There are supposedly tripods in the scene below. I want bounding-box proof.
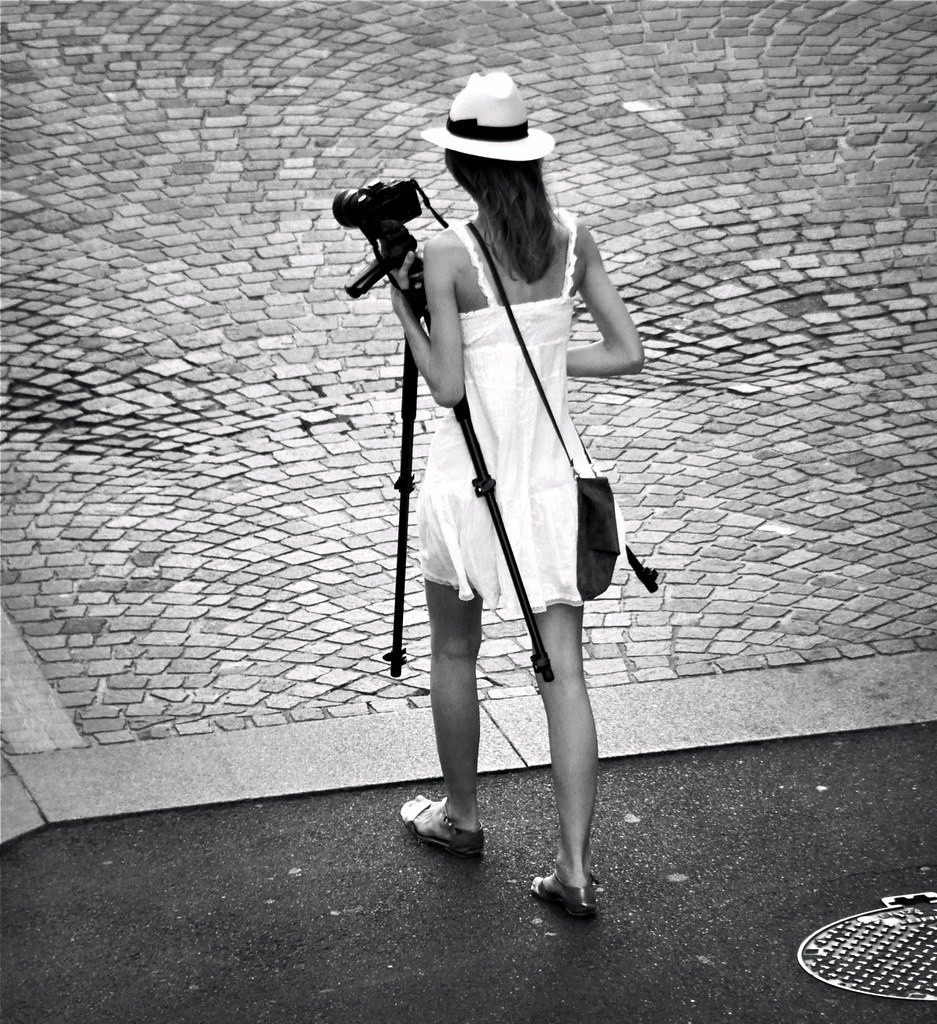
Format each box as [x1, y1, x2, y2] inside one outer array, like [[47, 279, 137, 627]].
[[345, 219, 658, 684]]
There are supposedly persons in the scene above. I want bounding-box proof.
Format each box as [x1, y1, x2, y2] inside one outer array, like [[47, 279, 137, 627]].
[[391, 71, 642, 919]]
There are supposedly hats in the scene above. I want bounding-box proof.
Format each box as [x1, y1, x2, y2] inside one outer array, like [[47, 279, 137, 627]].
[[420, 71, 555, 162]]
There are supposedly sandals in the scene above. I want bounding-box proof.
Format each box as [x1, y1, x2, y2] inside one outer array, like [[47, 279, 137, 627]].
[[401, 796, 483, 857], [532, 869, 601, 913]]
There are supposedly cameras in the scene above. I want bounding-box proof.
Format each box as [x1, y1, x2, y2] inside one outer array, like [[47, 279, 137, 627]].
[[331, 178, 423, 240]]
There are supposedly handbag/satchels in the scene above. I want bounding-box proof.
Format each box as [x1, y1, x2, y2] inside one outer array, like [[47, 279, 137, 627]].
[[577, 477, 621, 601]]
